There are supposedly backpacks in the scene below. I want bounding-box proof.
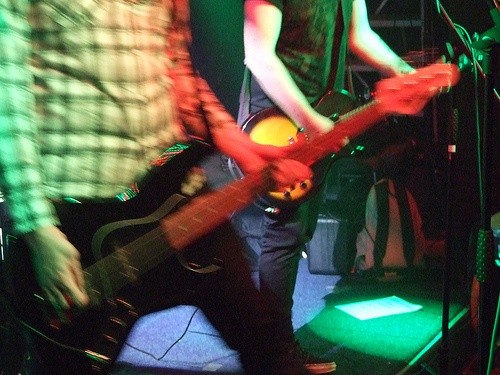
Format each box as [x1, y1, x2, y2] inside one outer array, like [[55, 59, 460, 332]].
[[353, 175, 427, 276]]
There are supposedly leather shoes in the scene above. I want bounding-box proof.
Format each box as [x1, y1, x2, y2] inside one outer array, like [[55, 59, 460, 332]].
[[287, 343, 337, 373]]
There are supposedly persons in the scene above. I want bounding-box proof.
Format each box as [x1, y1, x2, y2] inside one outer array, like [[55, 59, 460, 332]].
[[355, 173, 447, 285], [236, 0, 417, 375], [0, 0, 313, 375], [470, 212, 500, 346]]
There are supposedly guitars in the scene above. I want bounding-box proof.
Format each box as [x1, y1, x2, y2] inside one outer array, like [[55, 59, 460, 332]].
[[0, 64, 460, 375], [228, 56, 452, 214]]
